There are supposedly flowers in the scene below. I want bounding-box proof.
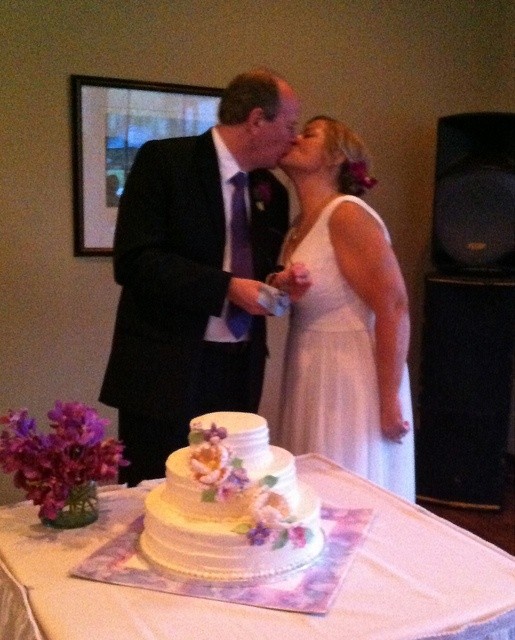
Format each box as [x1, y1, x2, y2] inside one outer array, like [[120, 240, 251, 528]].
[[0, 399, 131, 521], [187, 422, 314, 552]]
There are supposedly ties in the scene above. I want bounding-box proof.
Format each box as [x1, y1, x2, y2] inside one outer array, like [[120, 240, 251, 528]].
[[225, 171, 256, 340]]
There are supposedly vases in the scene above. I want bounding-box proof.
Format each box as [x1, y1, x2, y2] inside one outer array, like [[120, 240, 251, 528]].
[[45, 480, 99, 528]]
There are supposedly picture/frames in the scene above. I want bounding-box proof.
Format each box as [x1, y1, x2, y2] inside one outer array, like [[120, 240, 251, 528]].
[[69, 73, 226, 255]]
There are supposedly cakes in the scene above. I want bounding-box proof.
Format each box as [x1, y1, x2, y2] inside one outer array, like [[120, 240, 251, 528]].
[[137, 411, 328, 583]]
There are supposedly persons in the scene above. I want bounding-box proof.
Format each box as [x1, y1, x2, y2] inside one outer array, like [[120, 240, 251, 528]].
[[277, 115, 416, 506], [98, 69, 303, 487]]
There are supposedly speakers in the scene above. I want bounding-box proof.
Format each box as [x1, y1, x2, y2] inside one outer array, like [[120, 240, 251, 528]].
[[428, 110, 514, 276], [414, 272, 515, 514]]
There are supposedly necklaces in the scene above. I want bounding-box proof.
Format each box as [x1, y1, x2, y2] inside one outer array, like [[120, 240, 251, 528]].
[[292, 193, 337, 241]]
